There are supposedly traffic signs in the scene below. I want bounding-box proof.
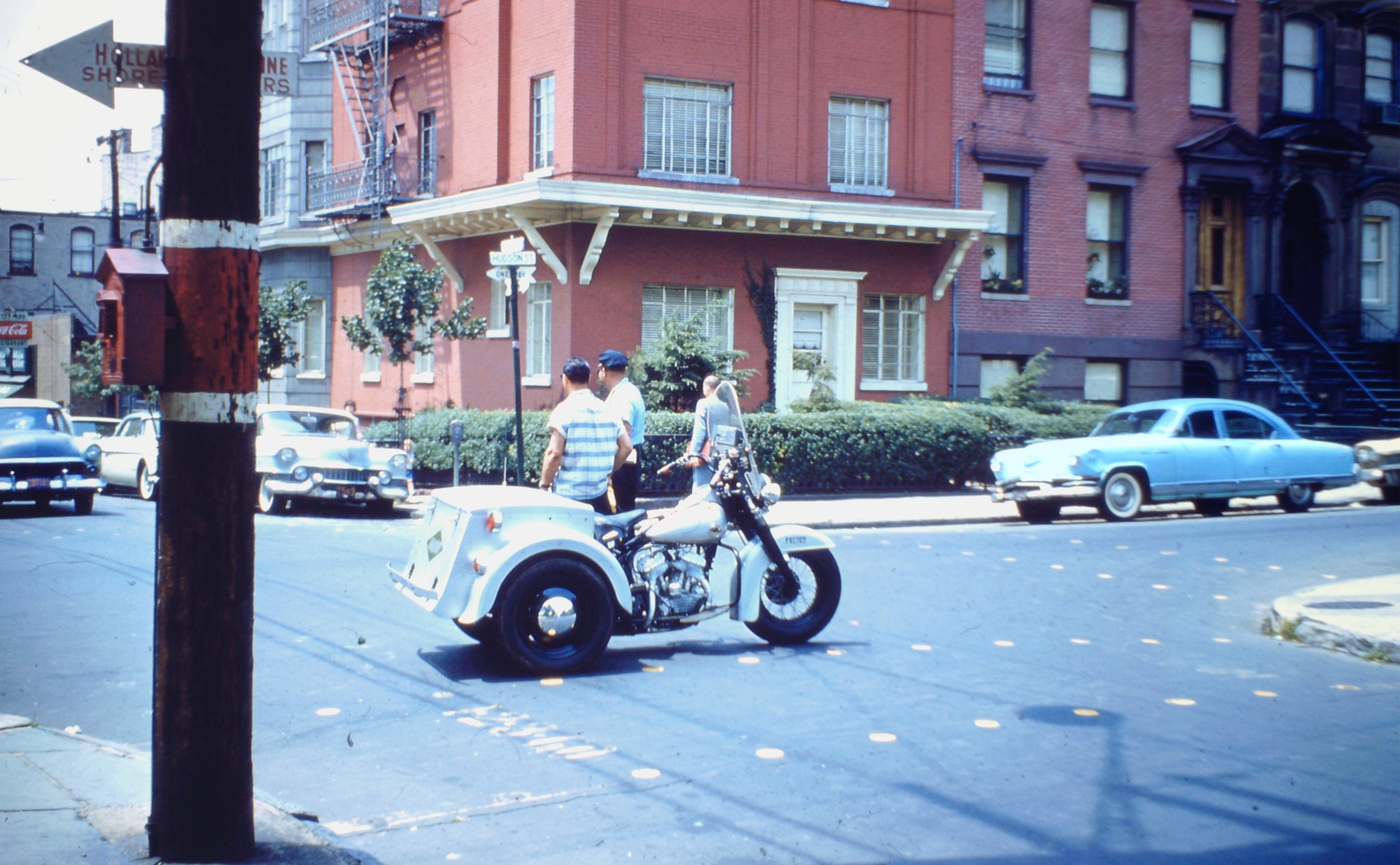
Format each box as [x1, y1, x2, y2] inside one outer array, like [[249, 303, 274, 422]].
[[486, 265, 537, 285], [19, 19, 302, 109]]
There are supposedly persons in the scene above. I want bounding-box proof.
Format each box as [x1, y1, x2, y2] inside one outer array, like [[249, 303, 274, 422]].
[[330, 399, 363, 439], [540, 356, 633, 540], [689, 375, 730, 493], [400, 438, 416, 501], [598, 349, 645, 547]]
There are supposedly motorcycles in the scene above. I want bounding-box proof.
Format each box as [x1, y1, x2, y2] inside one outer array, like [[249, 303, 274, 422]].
[[387, 378, 842, 679]]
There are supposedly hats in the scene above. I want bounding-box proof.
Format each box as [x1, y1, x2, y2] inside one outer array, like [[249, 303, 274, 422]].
[[598, 349, 628, 369]]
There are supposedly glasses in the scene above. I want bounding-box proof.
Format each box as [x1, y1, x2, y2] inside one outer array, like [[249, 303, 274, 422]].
[[560, 372, 565, 380]]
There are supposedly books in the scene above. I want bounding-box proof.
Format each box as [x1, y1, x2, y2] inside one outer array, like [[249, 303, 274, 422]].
[[683, 439, 711, 467]]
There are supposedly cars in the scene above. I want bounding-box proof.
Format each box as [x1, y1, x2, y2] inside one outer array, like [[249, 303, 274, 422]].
[[250, 404, 412, 513], [0, 397, 162, 516], [988, 398, 1399, 523]]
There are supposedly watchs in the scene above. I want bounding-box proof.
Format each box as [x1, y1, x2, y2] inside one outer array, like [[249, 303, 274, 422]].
[[539, 481, 550, 488]]
